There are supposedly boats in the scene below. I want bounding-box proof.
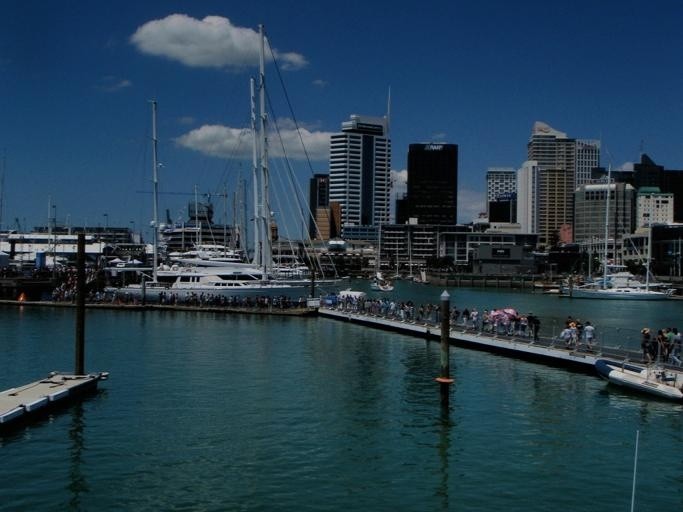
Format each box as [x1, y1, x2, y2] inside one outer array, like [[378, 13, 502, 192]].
[[594, 357, 683, 401], [367, 276, 396, 293]]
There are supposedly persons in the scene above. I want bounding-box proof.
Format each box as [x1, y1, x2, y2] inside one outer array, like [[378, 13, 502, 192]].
[[52, 266, 134, 306], [341, 295, 539, 341], [559, 317, 594, 352], [642, 327, 682, 368], [159, 291, 312, 310]]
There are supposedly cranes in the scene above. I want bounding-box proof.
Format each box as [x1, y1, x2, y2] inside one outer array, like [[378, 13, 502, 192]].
[[129, 180, 229, 203]]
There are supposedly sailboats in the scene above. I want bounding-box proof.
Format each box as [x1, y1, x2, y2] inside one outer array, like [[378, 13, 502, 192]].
[[103, 20, 350, 302], [567, 161, 671, 303], [0, 194, 112, 268]]
